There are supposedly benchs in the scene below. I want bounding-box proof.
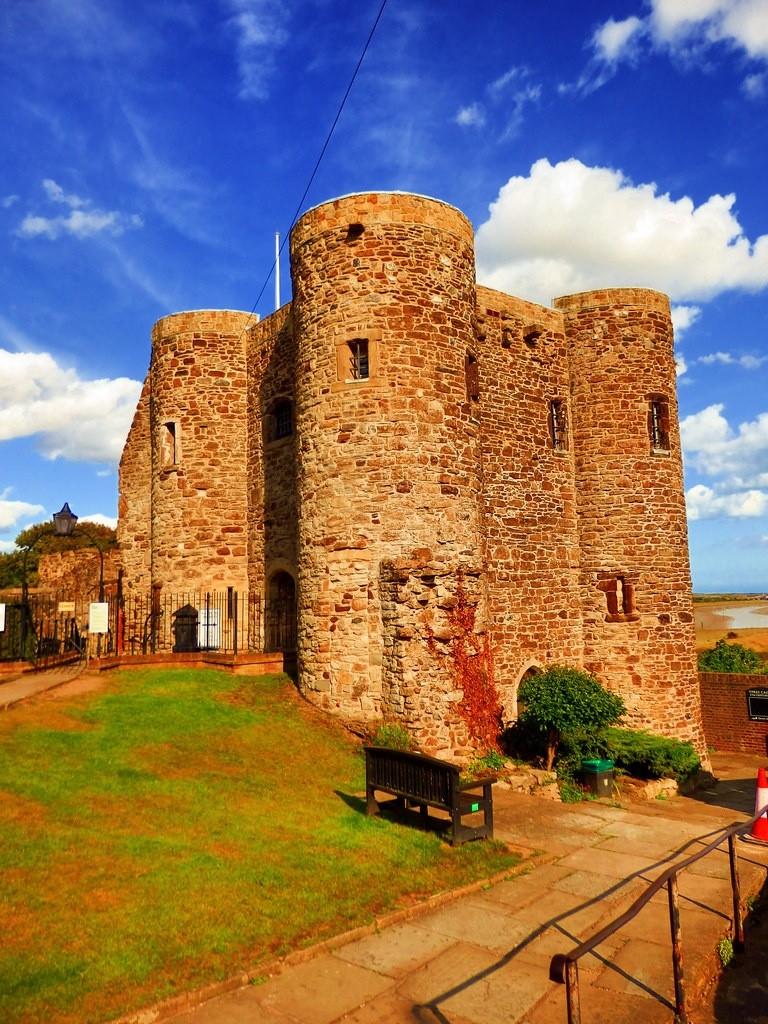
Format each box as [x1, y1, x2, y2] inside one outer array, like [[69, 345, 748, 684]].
[[364, 746, 499, 848]]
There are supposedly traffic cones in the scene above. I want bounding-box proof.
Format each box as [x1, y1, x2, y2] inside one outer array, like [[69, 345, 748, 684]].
[[738, 767, 768, 846]]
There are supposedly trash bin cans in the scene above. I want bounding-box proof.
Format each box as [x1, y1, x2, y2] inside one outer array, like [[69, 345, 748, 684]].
[[86, 624, 110, 655], [171, 604, 201, 652], [583, 759, 616, 799]]
